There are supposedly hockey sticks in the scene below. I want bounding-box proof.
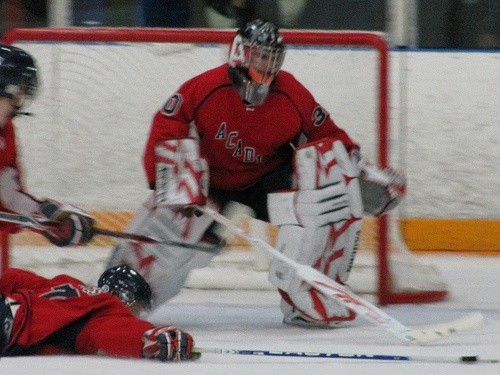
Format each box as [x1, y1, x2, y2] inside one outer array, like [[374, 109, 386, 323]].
[[192, 348, 500, 363], [0, 209, 225, 257], [192, 205, 483, 346]]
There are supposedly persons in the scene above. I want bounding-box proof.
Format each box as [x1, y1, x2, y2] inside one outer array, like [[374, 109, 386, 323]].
[[105, 18, 406, 329], [0, 44, 199, 361]]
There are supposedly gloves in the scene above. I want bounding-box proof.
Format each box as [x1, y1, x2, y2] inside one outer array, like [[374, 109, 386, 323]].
[[139, 323, 192, 361], [32, 198, 95, 245]]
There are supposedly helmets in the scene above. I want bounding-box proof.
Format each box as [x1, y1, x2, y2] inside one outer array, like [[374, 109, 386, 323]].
[[227, 19, 284, 105], [0, 43, 37, 87], [97, 264, 151, 306]]
